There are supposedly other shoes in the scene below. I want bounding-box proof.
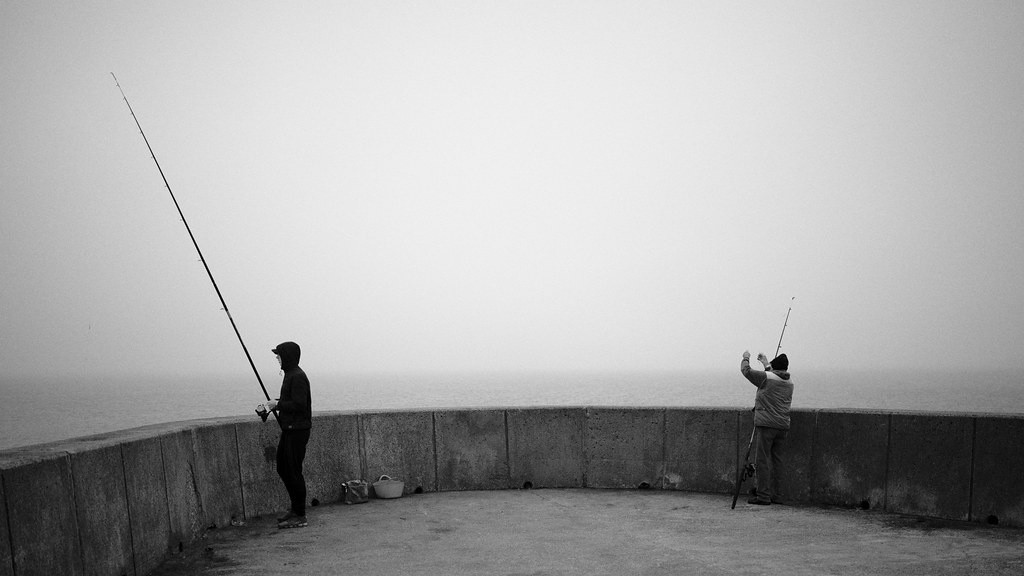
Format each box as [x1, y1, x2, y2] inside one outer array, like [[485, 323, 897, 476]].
[[747, 497, 770, 505]]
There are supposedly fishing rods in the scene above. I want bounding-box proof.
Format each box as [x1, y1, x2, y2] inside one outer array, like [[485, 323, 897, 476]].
[[731, 295, 795, 509], [110, 71, 283, 431]]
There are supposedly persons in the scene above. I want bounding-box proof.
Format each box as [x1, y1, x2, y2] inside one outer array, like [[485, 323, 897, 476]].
[[267, 341, 312, 526], [742, 349, 794, 505]]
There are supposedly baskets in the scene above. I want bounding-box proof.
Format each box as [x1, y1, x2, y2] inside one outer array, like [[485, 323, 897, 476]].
[[373, 474, 404, 498]]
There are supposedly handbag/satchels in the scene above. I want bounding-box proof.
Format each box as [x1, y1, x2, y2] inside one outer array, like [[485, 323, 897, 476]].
[[343, 480, 369, 504]]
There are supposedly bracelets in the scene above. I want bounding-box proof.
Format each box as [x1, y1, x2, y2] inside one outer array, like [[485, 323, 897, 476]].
[[276, 401, 279, 410]]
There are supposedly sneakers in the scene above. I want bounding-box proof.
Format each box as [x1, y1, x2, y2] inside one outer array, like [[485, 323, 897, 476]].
[[278, 515, 308, 528], [277, 511, 293, 521]]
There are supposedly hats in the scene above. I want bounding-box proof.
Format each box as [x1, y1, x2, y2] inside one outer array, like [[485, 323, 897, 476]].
[[770, 354, 788, 370], [272, 348, 279, 355]]
